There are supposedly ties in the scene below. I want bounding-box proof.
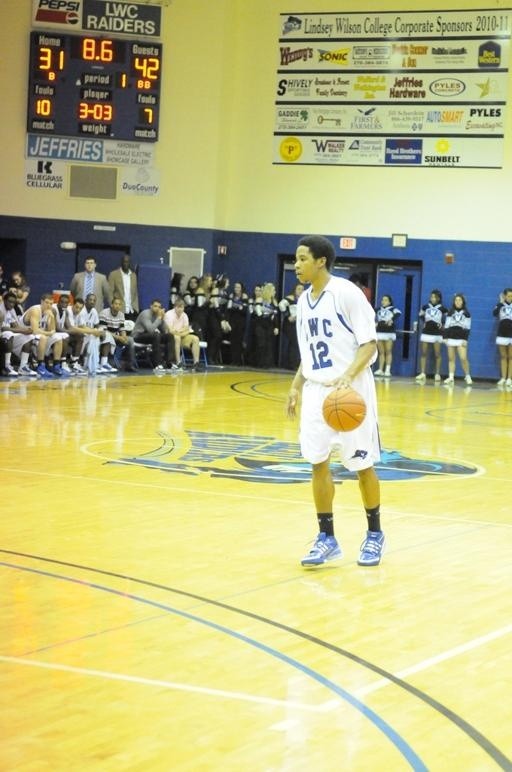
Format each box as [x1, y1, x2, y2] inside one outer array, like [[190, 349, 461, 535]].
[[85, 276, 92, 296]]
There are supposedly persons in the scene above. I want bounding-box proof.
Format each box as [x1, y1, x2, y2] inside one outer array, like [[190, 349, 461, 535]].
[[415, 290, 450, 381], [287, 236, 385, 567], [2, 255, 305, 376], [374, 294, 402, 377], [492, 288, 512, 386], [349, 273, 372, 304], [442, 294, 473, 385]]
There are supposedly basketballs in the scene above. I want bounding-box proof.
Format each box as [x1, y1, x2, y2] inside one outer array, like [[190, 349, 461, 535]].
[[324, 388, 364, 431]]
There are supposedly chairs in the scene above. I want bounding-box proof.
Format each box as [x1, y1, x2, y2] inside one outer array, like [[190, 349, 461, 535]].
[[111, 320, 210, 376]]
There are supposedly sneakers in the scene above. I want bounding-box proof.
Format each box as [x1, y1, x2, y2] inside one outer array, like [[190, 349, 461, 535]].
[[374, 367, 511, 386], [3, 361, 205, 376], [358, 531, 384, 566], [301, 531, 342, 568]]
